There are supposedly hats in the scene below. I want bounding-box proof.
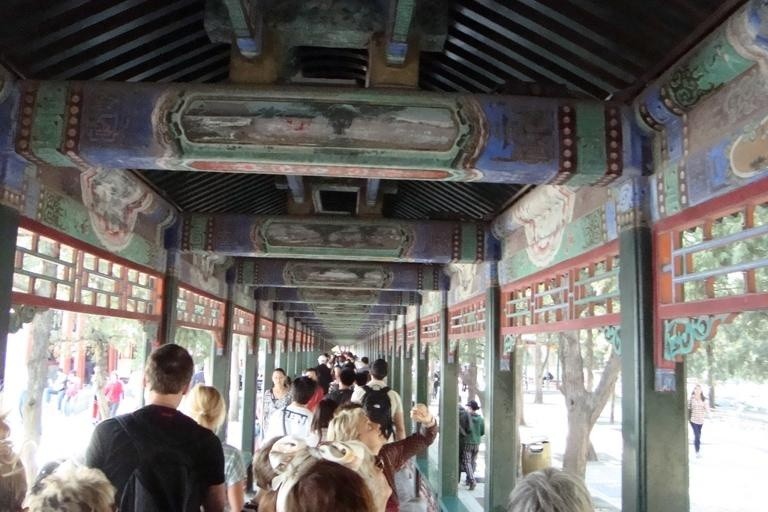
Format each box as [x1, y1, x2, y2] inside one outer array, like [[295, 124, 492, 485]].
[[466, 400, 478, 410]]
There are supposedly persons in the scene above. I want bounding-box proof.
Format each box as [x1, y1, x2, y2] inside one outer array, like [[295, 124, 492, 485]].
[[503, 467, 595, 512], [688, 385, 711, 458]]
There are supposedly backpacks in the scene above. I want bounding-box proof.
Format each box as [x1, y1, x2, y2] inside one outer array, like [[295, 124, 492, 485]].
[[116, 414, 203, 512], [361, 386, 391, 440]]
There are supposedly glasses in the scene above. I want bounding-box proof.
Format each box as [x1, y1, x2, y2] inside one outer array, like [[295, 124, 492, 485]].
[[371, 456, 384, 471]]
